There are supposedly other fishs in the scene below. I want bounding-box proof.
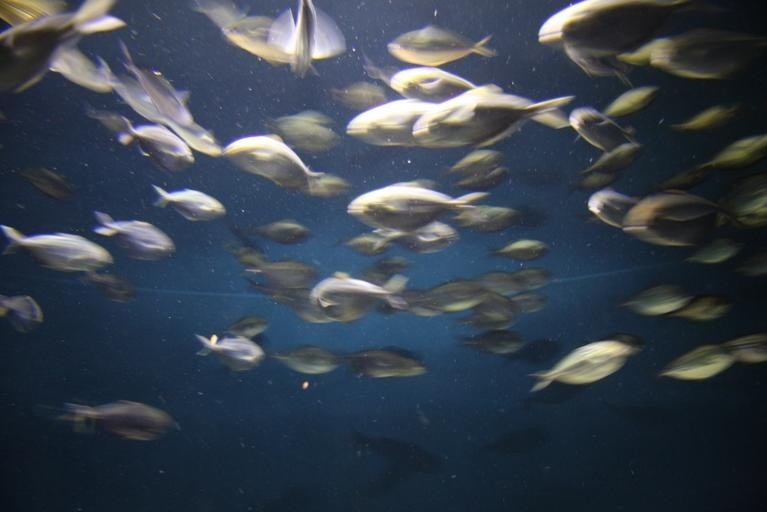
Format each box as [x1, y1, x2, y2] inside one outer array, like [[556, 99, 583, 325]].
[[55, 400, 183, 443], [0, 0, 767, 393]]
